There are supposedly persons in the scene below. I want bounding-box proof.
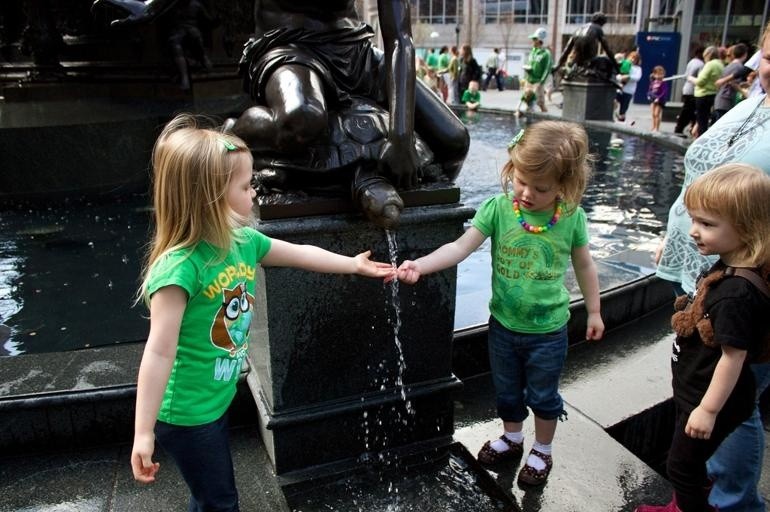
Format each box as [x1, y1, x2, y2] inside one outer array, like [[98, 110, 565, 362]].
[[629, 25, 770, 512], [128, 112, 397, 512], [219, 1, 469, 191], [383, 119, 606, 489], [371, 41, 385, 63], [669, 162, 770, 510], [552, 10, 621, 73]]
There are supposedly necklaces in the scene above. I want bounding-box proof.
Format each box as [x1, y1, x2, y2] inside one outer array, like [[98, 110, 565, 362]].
[[511, 194, 565, 234]]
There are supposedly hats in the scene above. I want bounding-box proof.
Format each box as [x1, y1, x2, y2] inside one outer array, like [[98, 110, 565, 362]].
[[614, 53, 625, 64], [528, 29, 546, 43]]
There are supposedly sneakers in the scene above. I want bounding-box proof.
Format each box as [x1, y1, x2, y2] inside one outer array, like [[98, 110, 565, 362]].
[[479, 436, 524, 463], [520, 448, 553, 485], [634, 491, 680, 512]]
